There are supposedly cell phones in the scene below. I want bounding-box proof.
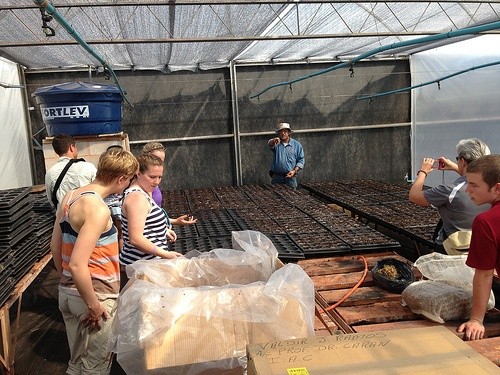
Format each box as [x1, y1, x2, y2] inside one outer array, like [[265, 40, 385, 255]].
[[431, 159, 443, 168]]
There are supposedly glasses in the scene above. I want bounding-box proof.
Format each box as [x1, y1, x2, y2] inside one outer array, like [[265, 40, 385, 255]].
[[456, 156, 463, 161]]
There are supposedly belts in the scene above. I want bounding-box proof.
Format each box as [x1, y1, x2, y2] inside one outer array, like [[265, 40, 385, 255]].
[[276, 172, 297, 176]]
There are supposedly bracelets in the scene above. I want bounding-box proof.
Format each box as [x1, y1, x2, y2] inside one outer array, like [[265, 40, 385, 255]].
[[416, 170, 428, 177]]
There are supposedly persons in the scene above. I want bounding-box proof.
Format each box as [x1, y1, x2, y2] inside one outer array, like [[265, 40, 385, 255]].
[[268, 122, 305, 190], [100, 144, 131, 253], [50, 145, 141, 375], [45, 134, 99, 214], [407, 137, 491, 264], [456, 154, 500, 341], [119, 151, 185, 288], [143, 141, 197, 226]]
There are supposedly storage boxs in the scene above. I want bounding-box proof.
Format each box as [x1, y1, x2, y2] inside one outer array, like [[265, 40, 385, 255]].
[[246, 326, 500, 375], [140, 257, 306, 375]]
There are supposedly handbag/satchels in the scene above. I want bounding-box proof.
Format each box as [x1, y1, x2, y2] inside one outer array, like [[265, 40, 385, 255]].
[[443, 230, 472, 255]]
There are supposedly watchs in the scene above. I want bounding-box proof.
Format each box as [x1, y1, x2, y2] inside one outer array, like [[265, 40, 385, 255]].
[[293, 169, 298, 173]]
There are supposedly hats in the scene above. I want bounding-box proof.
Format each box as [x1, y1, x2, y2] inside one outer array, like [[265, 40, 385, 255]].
[[275, 123, 292, 135]]
